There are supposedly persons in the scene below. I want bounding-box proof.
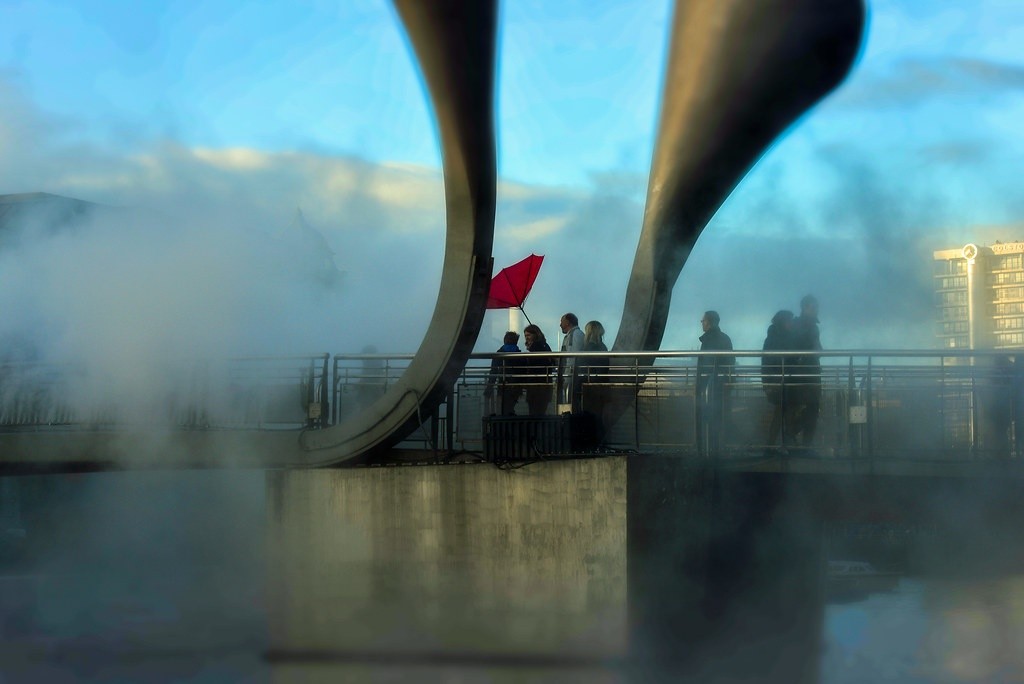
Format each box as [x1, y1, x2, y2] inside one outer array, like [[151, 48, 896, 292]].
[[761, 310, 806, 460], [522, 324, 556, 418], [353, 344, 386, 417], [482, 331, 526, 415], [695, 310, 736, 457], [581, 320, 611, 453], [558, 313, 584, 412], [793, 294, 824, 460]]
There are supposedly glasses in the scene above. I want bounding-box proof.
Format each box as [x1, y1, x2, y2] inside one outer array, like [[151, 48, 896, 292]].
[[701, 320, 705, 323]]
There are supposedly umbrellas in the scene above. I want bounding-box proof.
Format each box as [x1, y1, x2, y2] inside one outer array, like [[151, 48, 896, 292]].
[[486, 253, 546, 325]]
